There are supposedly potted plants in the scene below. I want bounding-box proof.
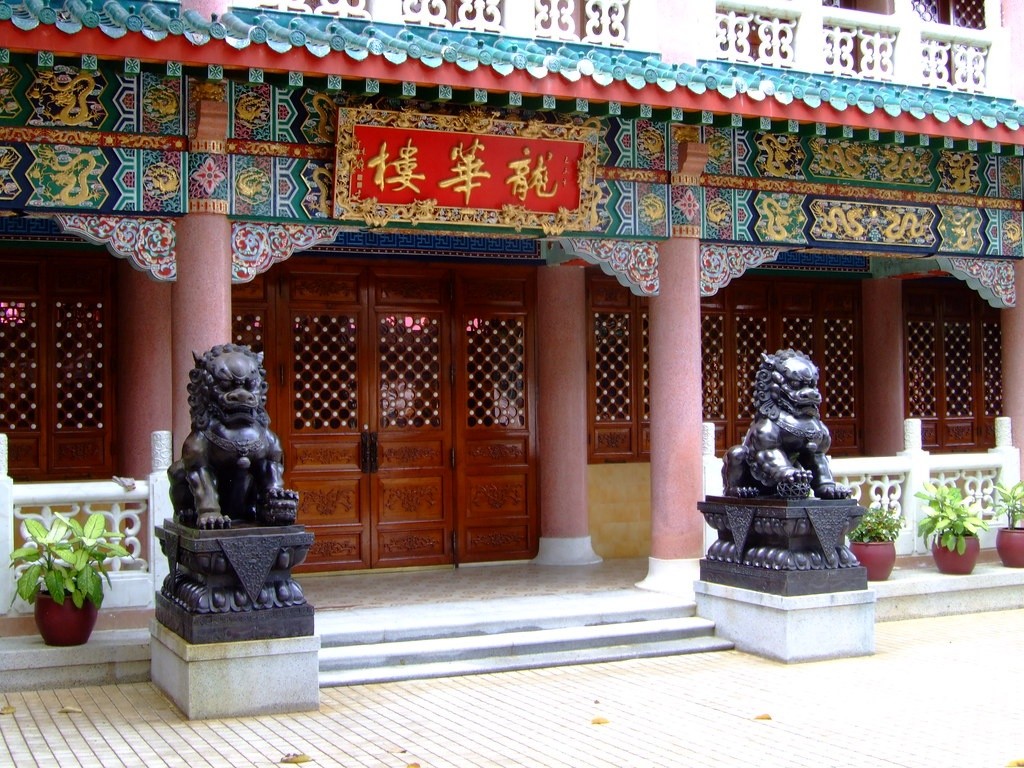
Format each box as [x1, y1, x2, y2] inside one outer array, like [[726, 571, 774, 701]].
[[985, 480, 1024, 568], [847, 506, 908, 580], [8, 511, 135, 648], [914, 480, 990, 575]]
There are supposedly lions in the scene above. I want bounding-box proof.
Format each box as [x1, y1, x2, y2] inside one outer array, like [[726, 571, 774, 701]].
[[721, 349, 853, 499], [168, 344, 299, 530]]
